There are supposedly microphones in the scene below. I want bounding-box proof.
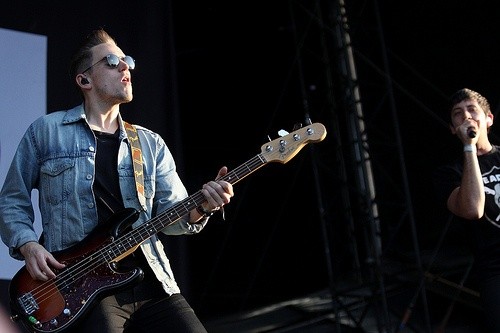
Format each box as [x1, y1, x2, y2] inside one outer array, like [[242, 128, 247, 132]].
[[467, 126, 476, 139]]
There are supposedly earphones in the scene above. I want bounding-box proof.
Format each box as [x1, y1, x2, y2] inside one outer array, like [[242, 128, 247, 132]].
[[81, 77, 89, 85]]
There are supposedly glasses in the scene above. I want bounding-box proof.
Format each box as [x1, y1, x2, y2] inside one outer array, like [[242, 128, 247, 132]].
[[81, 54, 135, 73]]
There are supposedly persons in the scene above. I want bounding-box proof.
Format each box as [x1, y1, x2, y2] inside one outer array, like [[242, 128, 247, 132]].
[[0, 31, 234, 333], [434, 88, 500, 333]]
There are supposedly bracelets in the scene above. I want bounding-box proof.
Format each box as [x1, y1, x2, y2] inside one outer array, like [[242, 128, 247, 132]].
[[196, 205, 212, 217], [463, 145, 478, 152]]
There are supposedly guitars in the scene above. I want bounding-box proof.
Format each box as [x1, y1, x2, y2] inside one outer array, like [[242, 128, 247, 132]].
[[7, 113, 326, 333]]
